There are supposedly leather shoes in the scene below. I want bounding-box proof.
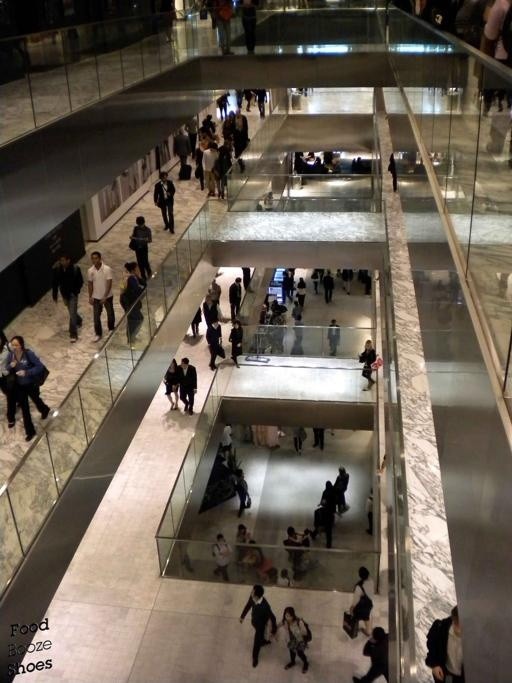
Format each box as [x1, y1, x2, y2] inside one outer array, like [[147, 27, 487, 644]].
[[9, 422, 15, 427], [42, 408, 50, 419], [26, 432, 35, 441], [185, 403, 193, 415]]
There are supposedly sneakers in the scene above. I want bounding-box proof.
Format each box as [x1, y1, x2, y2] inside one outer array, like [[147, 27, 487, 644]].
[[285, 662, 308, 673], [70, 337, 76, 342], [93, 335, 100, 342]]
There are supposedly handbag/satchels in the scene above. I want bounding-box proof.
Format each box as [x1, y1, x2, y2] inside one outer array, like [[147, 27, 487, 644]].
[[343, 595, 372, 639], [33, 365, 49, 386], [302, 623, 311, 641]]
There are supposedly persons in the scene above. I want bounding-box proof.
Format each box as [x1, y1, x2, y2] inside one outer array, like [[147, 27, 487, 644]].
[[191, 265, 369, 370], [129, 215, 153, 281], [51, 252, 85, 342], [1, 328, 21, 428], [5, 334, 51, 441], [200, 0, 235, 55], [234, 0, 261, 55], [349, 566, 374, 637], [172, 357, 199, 416], [392, 0, 511, 168], [119, 260, 148, 345], [238, 584, 278, 667], [351, 626, 389, 682], [424, 604, 464, 683], [154, 170, 176, 233], [211, 463, 351, 588], [219, 423, 233, 460], [312, 427, 325, 449], [173, 89, 268, 198], [270, 605, 312, 672], [358, 339, 377, 391], [362, 488, 373, 535], [162, 358, 180, 409], [292, 426, 307, 456], [87, 250, 116, 342]]
